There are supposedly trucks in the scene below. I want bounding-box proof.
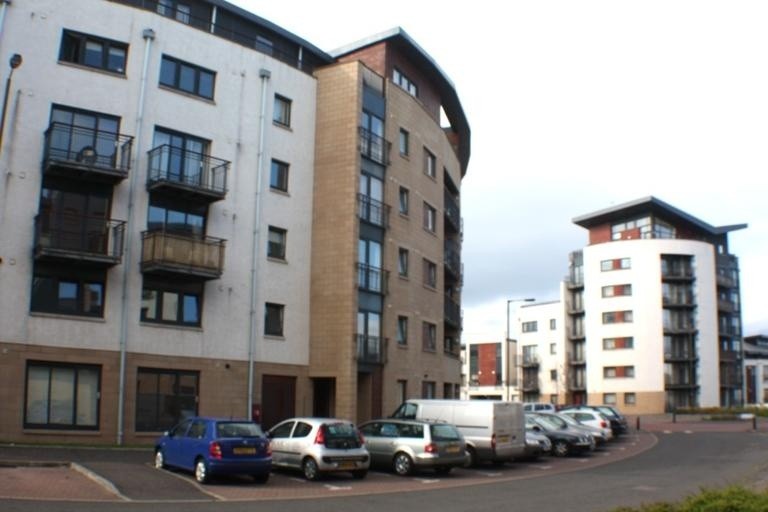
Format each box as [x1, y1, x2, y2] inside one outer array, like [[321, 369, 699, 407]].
[[387, 399, 526, 469]]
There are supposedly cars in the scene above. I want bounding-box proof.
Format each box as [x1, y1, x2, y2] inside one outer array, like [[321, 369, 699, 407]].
[[161, 409, 197, 431], [153, 416, 272, 484], [28, 398, 88, 424], [507, 401, 628, 462], [263, 417, 371, 480]]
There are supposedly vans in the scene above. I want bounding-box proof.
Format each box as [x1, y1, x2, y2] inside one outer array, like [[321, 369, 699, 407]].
[[356, 417, 466, 476]]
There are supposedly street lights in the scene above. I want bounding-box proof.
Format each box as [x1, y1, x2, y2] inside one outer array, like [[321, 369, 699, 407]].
[[0, 54, 22, 156], [507, 298, 535, 401]]
